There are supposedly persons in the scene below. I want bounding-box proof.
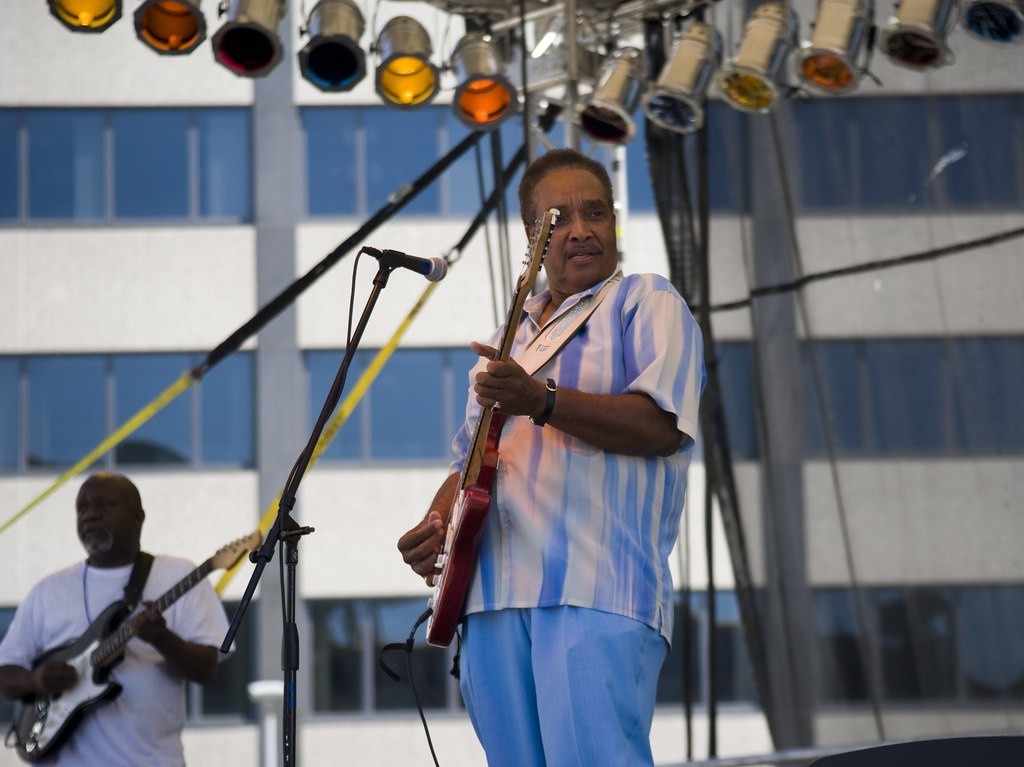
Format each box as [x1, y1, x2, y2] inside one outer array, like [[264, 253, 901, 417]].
[[0, 472, 235, 767], [398, 150, 704, 767]]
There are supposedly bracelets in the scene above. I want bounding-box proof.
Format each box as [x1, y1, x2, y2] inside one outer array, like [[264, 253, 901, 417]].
[[529, 379, 557, 425]]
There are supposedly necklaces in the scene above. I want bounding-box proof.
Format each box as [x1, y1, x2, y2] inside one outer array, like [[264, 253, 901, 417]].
[[84, 561, 93, 625]]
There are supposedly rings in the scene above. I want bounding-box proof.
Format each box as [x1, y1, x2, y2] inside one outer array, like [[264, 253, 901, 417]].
[[490, 402, 501, 412]]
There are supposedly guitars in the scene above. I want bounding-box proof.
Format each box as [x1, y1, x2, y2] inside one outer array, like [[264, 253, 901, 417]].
[[8, 528, 266, 766], [423, 207, 563, 650]]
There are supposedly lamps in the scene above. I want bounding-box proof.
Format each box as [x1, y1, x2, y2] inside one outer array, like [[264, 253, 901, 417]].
[[131, 0, 207, 57], [48, 0, 124, 33], [369, 0, 440, 110], [437, 1, 519, 131], [209, 0, 290, 80], [715, 0, 799, 116], [877, 1, 961, 75], [791, 1, 876, 95], [643, 0, 724, 135], [576, 17, 658, 145], [963, 0, 1024, 43], [297, 0, 368, 93]]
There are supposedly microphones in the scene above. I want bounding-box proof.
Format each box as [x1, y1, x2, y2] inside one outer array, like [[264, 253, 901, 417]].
[[361, 247, 448, 282]]
